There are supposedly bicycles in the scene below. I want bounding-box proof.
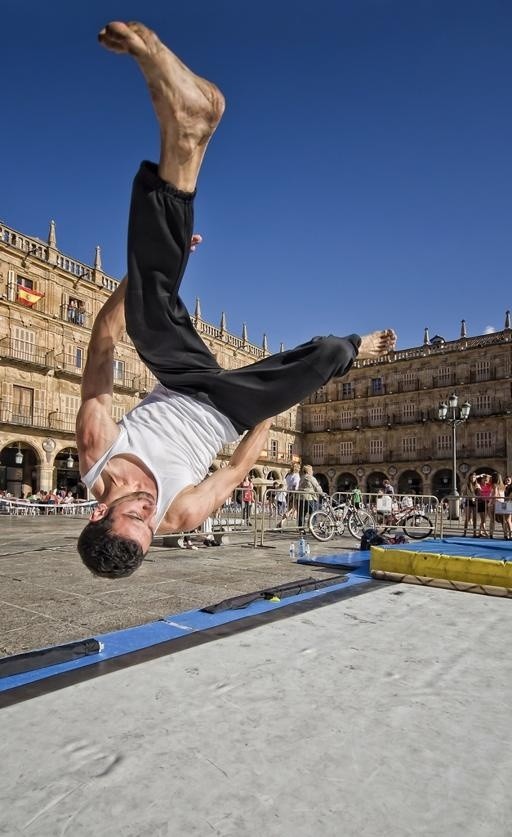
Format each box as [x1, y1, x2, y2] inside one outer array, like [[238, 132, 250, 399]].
[[306, 497, 435, 544]]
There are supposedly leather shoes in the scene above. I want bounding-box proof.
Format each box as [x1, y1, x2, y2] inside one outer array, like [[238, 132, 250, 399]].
[[177, 539, 222, 550]]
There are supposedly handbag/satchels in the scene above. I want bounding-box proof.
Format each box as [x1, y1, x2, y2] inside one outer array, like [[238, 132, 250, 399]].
[[496, 498, 512, 514], [361, 529, 409, 550]]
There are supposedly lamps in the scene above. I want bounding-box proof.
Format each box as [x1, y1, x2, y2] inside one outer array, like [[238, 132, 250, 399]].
[[67, 448, 74, 468], [15, 442, 23, 464]]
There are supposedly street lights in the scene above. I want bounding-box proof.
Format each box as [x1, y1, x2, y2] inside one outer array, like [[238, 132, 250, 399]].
[[436, 391, 474, 521]]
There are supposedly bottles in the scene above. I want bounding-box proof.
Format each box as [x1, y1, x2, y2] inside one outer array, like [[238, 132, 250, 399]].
[[289, 541, 295, 557], [298, 536, 305, 556], [305, 542, 310, 553]]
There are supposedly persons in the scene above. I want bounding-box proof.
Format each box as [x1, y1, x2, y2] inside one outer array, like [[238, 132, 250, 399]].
[[242, 476, 254, 526], [1, 488, 75, 515], [202, 463, 223, 547], [77, 20, 398, 577], [274, 462, 324, 535], [184, 531, 198, 550], [461, 471, 512, 540], [351, 486, 363, 509], [377, 480, 415, 519]]
